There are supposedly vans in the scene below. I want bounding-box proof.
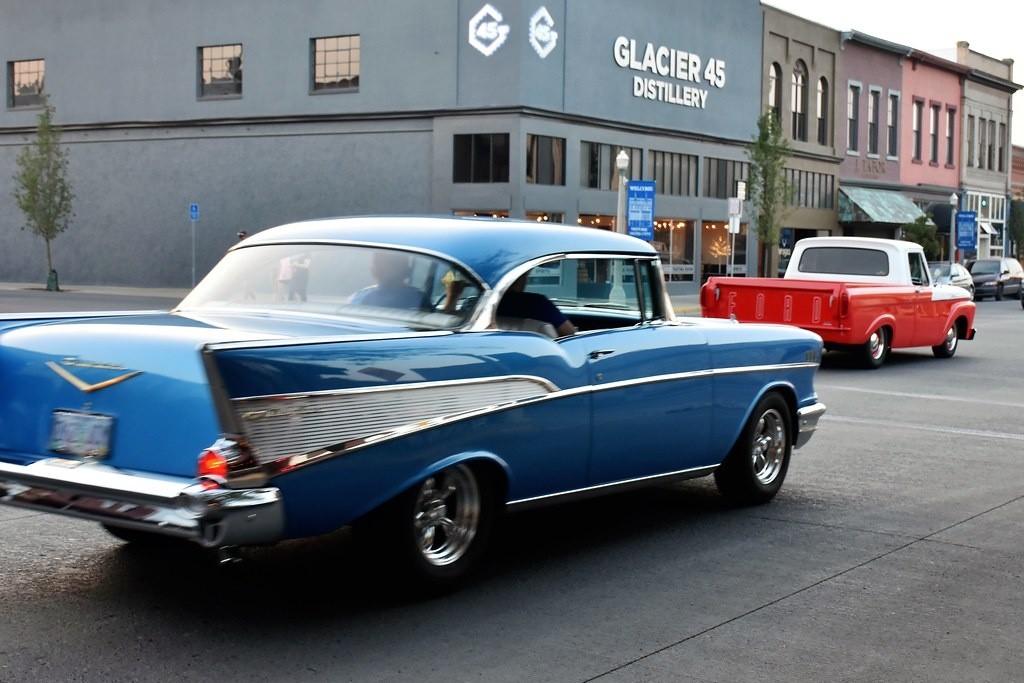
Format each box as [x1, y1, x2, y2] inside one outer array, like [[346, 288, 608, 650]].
[[964, 256, 1024, 301]]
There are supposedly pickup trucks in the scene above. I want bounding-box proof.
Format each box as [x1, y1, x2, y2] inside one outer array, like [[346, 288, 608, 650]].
[[700, 236, 978, 370]]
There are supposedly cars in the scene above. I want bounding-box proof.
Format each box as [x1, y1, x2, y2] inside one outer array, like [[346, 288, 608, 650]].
[[912, 259, 976, 303], [0, 211, 827, 594], [1018, 271, 1024, 310]]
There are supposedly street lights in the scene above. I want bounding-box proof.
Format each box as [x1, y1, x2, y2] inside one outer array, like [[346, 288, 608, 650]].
[[948, 192, 958, 262], [608, 149, 631, 305]]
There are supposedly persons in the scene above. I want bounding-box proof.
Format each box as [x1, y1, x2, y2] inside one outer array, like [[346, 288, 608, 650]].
[[236, 230, 316, 305], [346, 248, 464, 317], [462, 271, 577, 336]]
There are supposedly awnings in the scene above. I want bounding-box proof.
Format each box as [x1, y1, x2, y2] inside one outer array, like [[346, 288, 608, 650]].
[[979, 220, 998, 235], [837, 185, 935, 227]]
